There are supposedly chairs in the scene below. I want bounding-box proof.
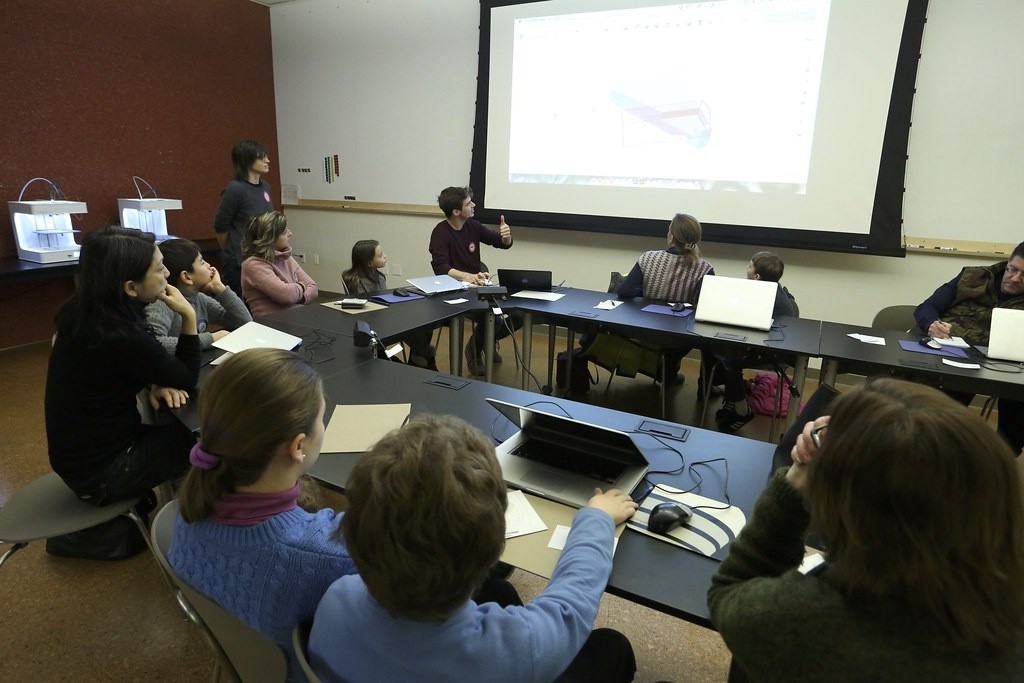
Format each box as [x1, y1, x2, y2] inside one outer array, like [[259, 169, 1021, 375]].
[[873, 306, 922, 334], [579, 270, 799, 443], [292, 626, 330, 681], [152, 498, 288, 683], [339, 266, 409, 364]]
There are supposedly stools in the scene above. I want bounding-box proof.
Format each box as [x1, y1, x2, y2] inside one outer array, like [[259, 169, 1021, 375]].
[[1, 469, 175, 597]]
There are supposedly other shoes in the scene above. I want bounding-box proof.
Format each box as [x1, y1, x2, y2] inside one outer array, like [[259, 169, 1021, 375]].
[[674, 373, 686, 383], [697, 386, 720, 399]]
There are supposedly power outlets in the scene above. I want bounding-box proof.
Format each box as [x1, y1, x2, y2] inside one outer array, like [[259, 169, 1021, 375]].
[[314, 254, 320, 264], [301, 252, 306, 263]]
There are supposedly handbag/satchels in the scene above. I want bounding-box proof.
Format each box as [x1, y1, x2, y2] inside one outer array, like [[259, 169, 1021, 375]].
[[45, 491, 157, 561], [557, 348, 590, 394]]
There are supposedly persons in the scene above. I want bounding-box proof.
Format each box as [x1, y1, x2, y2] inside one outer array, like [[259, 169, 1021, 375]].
[[166, 347, 359, 683], [307, 415, 639, 683], [619, 213, 715, 384], [240, 210, 318, 317], [344, 239, 439, 373], [913, 240, 1024, 458], [214, 140, 276, 300], [429, 185, 524, 375], [45, 227, 201, 561], [137, 239, 253, 430], [708, 376, 1024, 683], [715, 251, 795, 433]]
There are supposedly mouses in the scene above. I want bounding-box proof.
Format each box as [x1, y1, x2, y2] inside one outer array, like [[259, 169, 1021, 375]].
[[919, 336, 943, 349], [672, 303, 686, 312], [393, 288, 410, 297], [648, 503, 693, 534]]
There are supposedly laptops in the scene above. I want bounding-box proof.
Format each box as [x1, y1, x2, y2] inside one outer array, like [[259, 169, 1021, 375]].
[[405, 275, 469, 296], [210, 321, 303, 366], [496, 269, 552, 291], [974, 308, 1024, 362], [485, 398, 650, 509], [694, 275, 778, 330]]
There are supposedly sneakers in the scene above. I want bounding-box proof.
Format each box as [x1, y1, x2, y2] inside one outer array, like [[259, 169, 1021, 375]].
[[719, 404, 756, 432], [716, 400, 737, 419]]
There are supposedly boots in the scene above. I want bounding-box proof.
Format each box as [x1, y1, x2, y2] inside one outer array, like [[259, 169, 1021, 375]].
[[465, 334, 484, 375], [485, 341, 502, 362]]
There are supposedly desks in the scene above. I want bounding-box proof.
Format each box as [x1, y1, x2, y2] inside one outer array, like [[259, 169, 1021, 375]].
[[0, 240, 219, 303], [166, 284, 1024, 635]]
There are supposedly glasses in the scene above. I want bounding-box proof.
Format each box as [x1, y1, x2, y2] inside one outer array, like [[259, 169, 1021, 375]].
[[1008, 260, 1024, 276], [255, 152, 266, 159], [811, 424, 828, 449]]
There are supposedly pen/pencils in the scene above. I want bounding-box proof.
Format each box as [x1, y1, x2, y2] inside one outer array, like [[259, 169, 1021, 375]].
[[334, 301, 342, 305], [610, 299, 615, 305], [937, 317, 955, 342]]
